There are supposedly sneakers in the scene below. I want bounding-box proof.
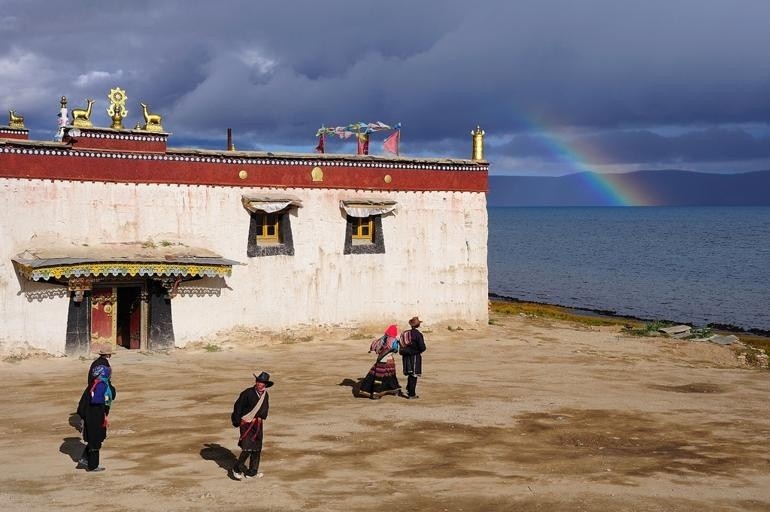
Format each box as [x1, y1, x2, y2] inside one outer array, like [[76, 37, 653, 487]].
[[231, 467, 264, 481], [78, 457, 105, 472], [372, 389, 419, 399]]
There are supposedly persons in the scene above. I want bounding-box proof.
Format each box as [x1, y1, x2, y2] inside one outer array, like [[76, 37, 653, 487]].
[[231, 371, 274, 480], [68, 343, 116, 472], [360, 324, 403, 400], [399, 316, 426, 399]]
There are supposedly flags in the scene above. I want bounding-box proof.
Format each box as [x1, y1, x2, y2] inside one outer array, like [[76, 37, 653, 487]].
[[384, 130, 398, 154], [316, 136, 324, 154]]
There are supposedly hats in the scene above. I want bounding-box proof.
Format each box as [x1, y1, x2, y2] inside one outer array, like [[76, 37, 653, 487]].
[[252, 372, 273, 387], [98, 344, 115, 355], [409, 317, 422, 327]]
